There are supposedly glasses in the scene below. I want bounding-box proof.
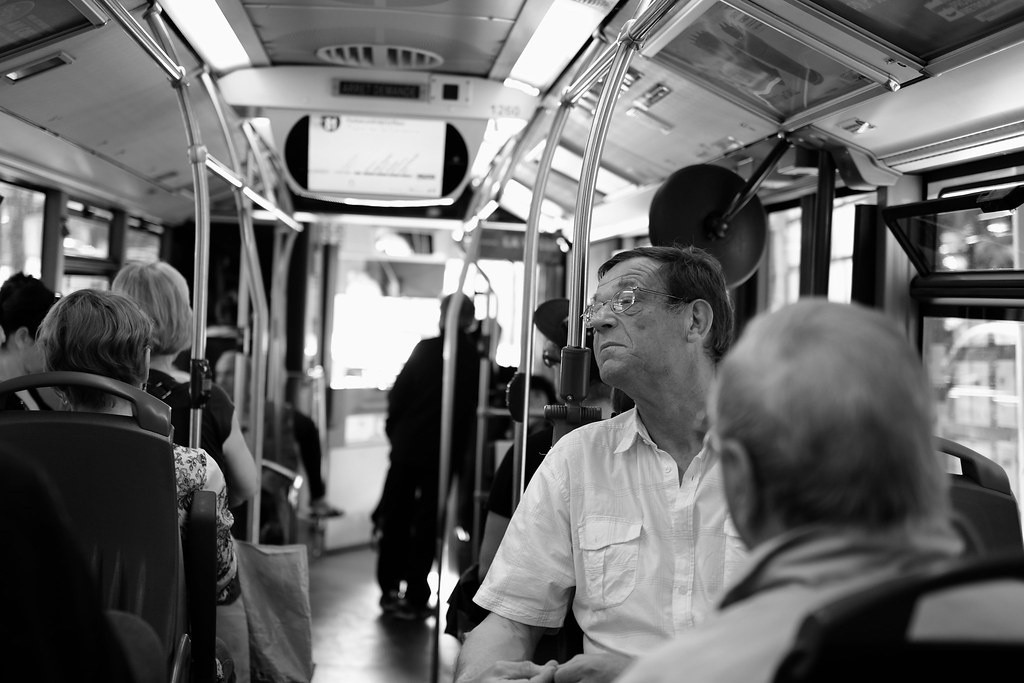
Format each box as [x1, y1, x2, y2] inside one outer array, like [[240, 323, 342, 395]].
[[581, 287, 691, 328]]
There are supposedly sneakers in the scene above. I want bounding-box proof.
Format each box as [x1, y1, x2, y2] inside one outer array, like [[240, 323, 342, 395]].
[[380, 590, 400, 610], [306, 502, 346, 517], [397, 602, 434, 621]]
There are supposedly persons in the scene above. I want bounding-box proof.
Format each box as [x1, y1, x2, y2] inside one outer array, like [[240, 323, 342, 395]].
[[33, 287, 242, 683], [606, 297, 1023, 682], [370, 289, 490, 624], [434, 313, 521, 579], [204, 349, 299, 548], [110, 256, 261, 510], [452, 239, 804, 682], [278, 364, 348, 524], [0, 270, 69, 422]]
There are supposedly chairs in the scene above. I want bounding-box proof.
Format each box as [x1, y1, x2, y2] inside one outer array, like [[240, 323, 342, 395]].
[[0, 326, 1022, 682]]
